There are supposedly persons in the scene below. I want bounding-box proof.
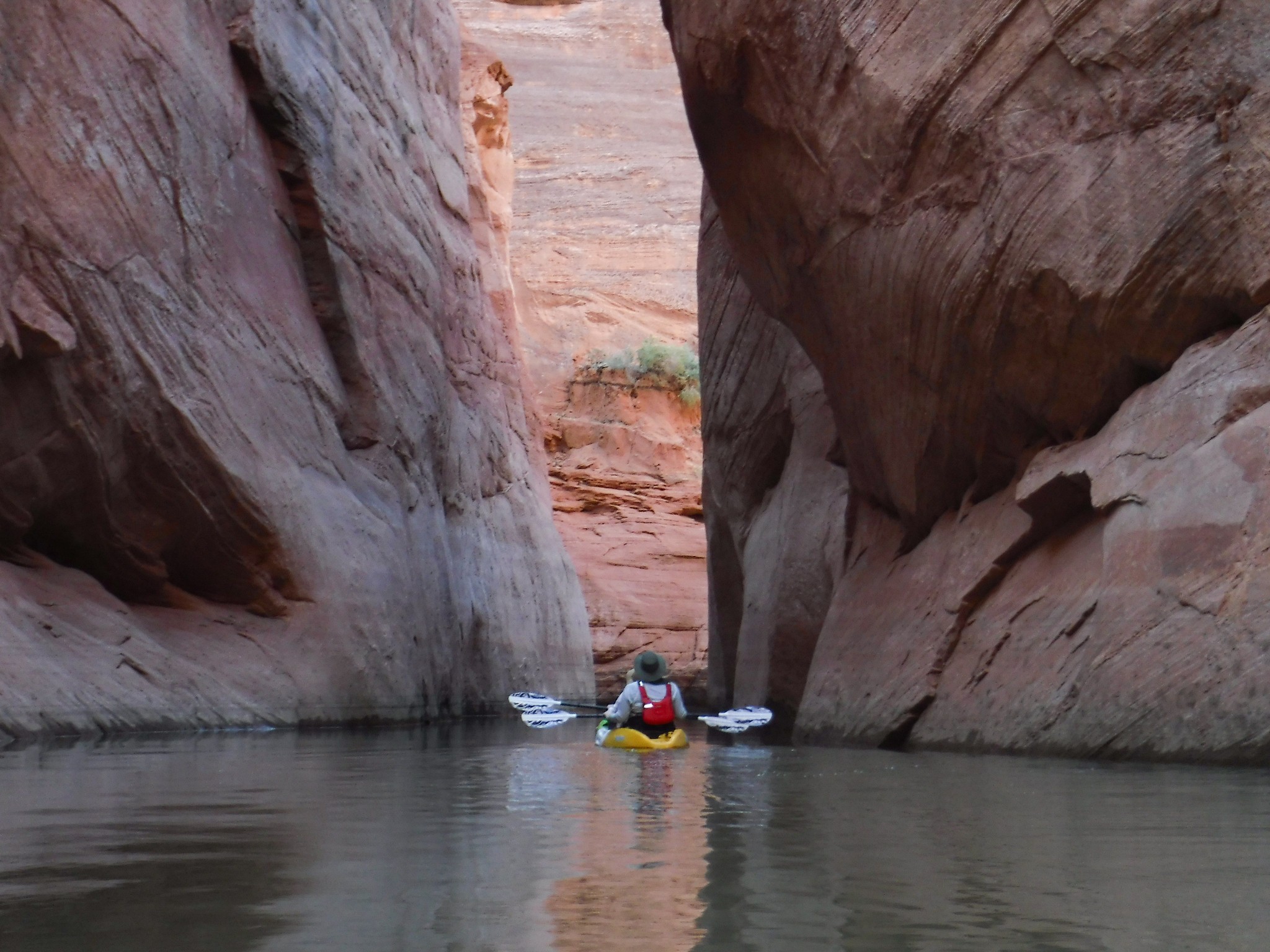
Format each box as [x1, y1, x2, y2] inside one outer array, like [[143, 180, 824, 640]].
[[603, 650, 687, 739]]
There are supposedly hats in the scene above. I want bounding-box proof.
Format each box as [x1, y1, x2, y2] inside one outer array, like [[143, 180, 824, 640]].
[[626, 669, 635, 684], [633, 651, 666, 681]]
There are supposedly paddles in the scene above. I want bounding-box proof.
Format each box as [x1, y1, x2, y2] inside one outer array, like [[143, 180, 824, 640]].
[[521, 709, 749, 733], [508, 691, 772, 726]]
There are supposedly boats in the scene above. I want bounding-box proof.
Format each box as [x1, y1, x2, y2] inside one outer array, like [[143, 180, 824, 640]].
[[594, 719, 690, 750]]
